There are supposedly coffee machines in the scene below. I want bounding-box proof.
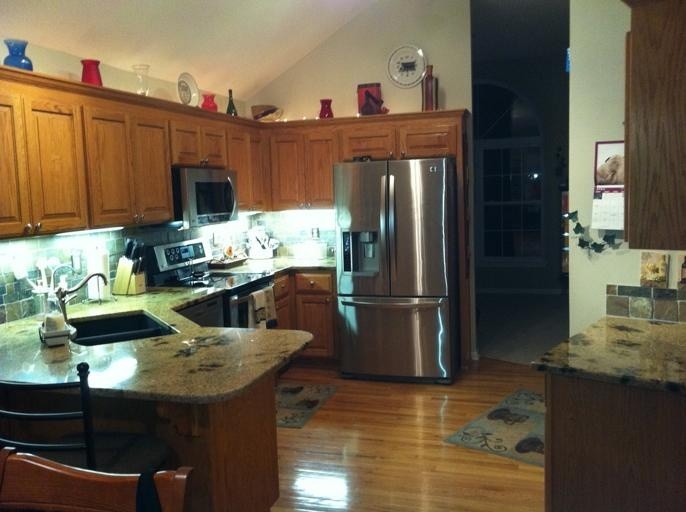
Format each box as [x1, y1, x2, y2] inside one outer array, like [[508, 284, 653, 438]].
[[246, 226, 275, 259]]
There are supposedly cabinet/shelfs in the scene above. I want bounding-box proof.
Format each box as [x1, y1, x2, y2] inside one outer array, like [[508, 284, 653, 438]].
[[622, 0, 686, 251], [0, 65, 87, 242], [293, 271, 335, 363], [268, 273, 293, 331], [268, 118, 340, 213], [229, 115, 267, 212], [86, 82, 170, 230], [341, 108, 467, 160], [170, 101, 228, 166]]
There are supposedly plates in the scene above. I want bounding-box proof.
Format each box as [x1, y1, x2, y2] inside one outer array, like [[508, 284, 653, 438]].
[[177, 72, 200, 107]]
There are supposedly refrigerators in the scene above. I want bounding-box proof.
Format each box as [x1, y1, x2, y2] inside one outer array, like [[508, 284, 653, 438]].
[[331, 154, 463, 386]]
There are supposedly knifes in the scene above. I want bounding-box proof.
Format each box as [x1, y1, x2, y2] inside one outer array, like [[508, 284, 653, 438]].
[[122, 236, 146, 274]]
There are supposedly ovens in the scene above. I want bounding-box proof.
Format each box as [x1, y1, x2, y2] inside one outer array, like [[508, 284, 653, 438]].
[[224, 284, 270, 328]]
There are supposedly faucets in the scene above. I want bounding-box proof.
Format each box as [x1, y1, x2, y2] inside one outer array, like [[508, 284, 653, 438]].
[[54, 272, 109, 325], [45, 264, 76, 314]]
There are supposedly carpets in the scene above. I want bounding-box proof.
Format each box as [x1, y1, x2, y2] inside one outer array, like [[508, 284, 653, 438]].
[[274, 377, 337, 428], [445, 387, 545, 478]]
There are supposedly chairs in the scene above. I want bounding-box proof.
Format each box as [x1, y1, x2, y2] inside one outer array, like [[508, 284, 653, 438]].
[[0, 362, 94, 472], [0, 444, 212, 512]]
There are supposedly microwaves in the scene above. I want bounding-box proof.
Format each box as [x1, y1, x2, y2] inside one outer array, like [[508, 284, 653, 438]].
[[172, 164, 240, 231]]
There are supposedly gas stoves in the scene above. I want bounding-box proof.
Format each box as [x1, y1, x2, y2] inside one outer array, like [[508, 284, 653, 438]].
[[156, 270, 273, 295]]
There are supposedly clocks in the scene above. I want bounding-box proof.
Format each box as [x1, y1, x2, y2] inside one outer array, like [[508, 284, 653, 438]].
[[385, 43, 426, 89]]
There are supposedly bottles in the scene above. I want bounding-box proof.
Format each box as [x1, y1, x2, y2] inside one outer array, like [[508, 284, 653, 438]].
[[226, 89, 238, 116], [682, 255, 686, 283], [420, 64, 440, 111], [318, 98, 334, 118], [201, 93, 217, 112], [2, 39, 32, 72], [79, 59, 102, 86]]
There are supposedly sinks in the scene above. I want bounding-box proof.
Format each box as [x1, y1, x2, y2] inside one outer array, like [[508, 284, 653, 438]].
[[65, 308, 164, 341], [71, 327, 181, 347]]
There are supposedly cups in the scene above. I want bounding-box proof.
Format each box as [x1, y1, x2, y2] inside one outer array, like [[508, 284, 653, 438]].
[[132, 65, 151, 97]]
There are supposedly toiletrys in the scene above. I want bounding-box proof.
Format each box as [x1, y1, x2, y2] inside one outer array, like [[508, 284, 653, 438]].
[[58, 275, 70, 306]]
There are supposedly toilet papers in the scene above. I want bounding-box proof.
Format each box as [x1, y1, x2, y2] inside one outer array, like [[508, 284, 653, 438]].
[[86, 248, 111, 303]]
